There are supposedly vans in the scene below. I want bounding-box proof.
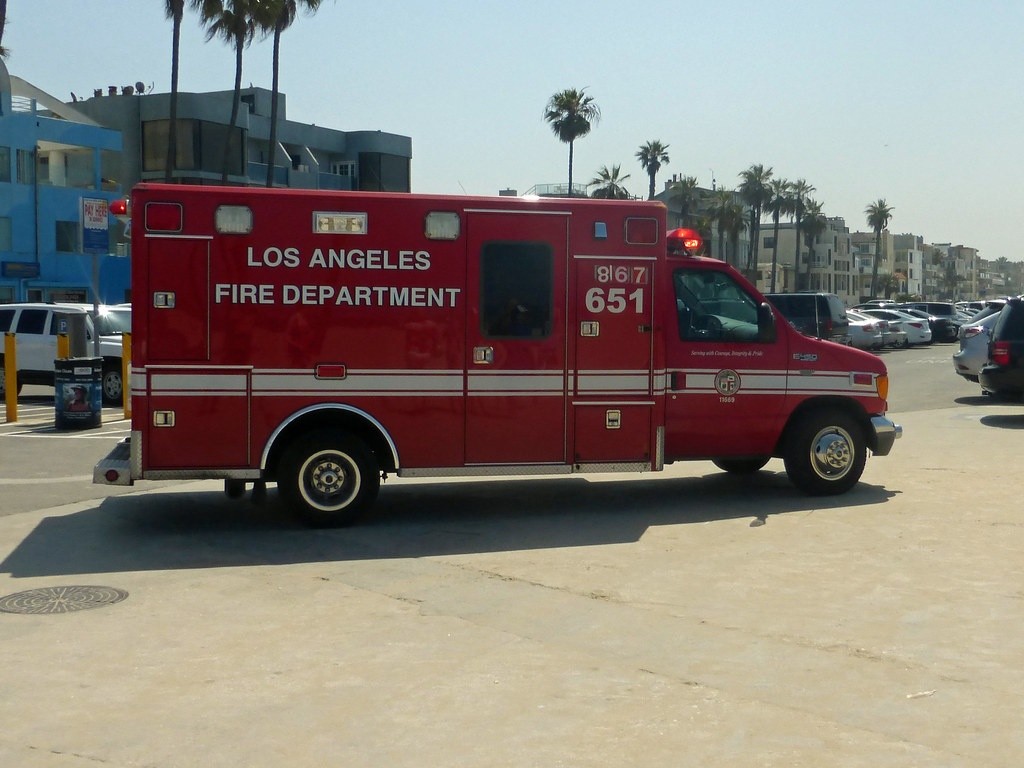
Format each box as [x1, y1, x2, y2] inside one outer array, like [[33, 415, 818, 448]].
[[760, 291, 850, 347]]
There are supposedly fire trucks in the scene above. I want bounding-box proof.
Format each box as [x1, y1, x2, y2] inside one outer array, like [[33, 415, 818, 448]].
[[92, 182, 903, 529]]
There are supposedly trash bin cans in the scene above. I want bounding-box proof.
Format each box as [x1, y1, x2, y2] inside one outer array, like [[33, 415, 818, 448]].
[[54, 357, 104, 429]]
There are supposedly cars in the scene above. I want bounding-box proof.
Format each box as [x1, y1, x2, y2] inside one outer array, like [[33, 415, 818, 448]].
[[843, 294, 1024, 404]]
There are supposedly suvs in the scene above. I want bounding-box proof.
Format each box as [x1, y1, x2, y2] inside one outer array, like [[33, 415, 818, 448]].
[[0, 300, 131, 406]]
